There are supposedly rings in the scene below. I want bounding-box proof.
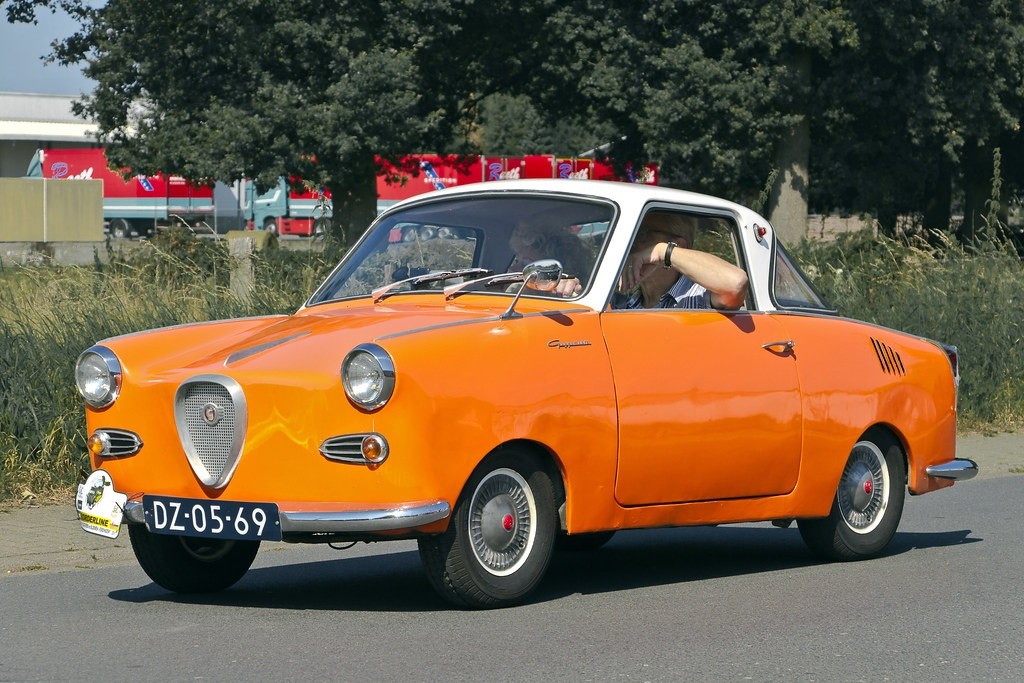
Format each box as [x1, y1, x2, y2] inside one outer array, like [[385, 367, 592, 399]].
[[627, 265, 632, 268]]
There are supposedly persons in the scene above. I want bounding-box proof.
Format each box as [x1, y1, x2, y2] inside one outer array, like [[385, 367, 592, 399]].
[[508, 216, 595, 289], [540, 211, 749, 311]]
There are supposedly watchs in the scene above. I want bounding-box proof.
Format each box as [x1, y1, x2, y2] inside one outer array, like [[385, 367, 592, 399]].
[[663, 242, 679, 270]]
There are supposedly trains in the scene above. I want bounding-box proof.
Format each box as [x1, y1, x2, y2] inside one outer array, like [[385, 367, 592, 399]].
[[23, 134, 662, 237]]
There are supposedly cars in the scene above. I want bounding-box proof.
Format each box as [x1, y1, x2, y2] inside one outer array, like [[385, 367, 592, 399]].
[[73, 180, 979, 613]]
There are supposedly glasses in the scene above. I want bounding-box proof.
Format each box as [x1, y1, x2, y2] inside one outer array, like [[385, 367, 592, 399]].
[[637, 228, 681, 243]]
